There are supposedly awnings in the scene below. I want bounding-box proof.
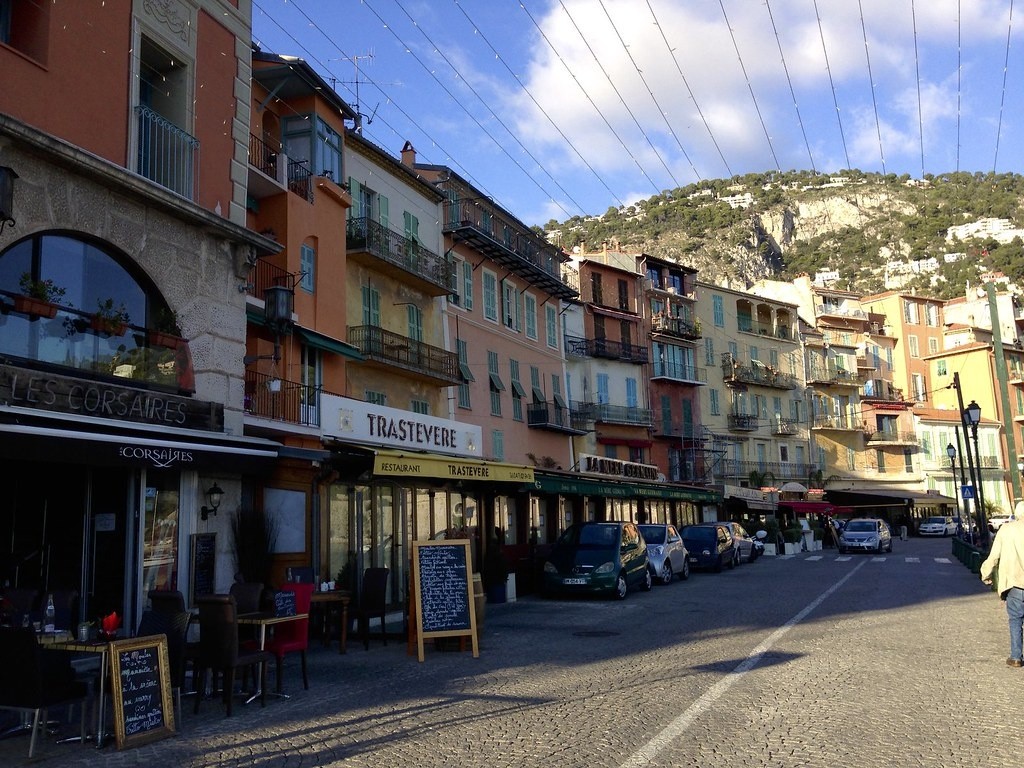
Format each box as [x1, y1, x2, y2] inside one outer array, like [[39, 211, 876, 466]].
[[524, 474, 723, 503], [346, 444, 535, 482], [730, 496, 778, 510], [825, 489, 958, 507], [296, 328, 367, 362], [775, 501, 853, 513]]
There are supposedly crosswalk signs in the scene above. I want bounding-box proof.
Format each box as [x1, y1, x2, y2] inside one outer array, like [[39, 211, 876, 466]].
[[961, 485, 974, 498]]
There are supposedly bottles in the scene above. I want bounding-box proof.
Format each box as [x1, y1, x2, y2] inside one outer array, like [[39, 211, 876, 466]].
[[23, 614, 29, 627], [46, 594, 55, 618]]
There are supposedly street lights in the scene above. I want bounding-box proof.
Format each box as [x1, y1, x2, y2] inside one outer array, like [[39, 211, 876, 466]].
[[962, 400, 989, 552], [946, 443, 963, 539]]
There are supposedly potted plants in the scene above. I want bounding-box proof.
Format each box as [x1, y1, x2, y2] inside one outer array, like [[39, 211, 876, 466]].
[[265, 376, 284, 394], [487, 539, 517, 603], [61, 297, 132, 341], [721, 356, 736, 379], [15, 273, 67, 320], [763, 520, 780, 556], [783, 529, 803, 555], [692, 318, 703, 339], [148, 309, 189, 350], [813, 527, 825, 551]]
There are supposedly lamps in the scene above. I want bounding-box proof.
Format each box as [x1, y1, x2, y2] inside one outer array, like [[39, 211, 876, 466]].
[[201, 483, 224, 522], [0, 165, 19, 236], [241, 281, 295, 365]]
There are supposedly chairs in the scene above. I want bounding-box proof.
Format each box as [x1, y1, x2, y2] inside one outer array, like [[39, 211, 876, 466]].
[[0, 657, 98, 758], [136, 566, 390, 716]]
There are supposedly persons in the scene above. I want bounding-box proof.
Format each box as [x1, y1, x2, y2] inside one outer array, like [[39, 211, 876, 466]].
[[900, 514, 909, 541], [980, 501, 1024, 667]]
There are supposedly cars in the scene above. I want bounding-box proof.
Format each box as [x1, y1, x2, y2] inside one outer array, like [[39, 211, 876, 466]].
[[917, 514, 1015, 538], [637, 524, 690, 586], [716, 521, 758, 567], [837, 518, 893, 555], [538, 520, 651, 601], [677, 522, 736, 573]]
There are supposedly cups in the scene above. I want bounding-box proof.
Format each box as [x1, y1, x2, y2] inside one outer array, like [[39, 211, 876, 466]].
[[321, 582, 328, 591], [328, 582, 335, 590]]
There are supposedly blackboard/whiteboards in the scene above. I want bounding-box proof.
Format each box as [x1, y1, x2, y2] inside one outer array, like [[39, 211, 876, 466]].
[[109, 634, 176, 753], [408, 539, 478, 637]]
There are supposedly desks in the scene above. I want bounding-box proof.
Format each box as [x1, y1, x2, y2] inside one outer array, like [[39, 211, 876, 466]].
[[43, 638, 111, 749], [236, 613, 310, 702], [312, 593, 351, 654]]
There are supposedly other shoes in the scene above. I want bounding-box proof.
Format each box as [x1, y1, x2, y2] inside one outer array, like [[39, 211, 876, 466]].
[[900, 537, 902, 541], [1006, 658, 1021, 667]]
